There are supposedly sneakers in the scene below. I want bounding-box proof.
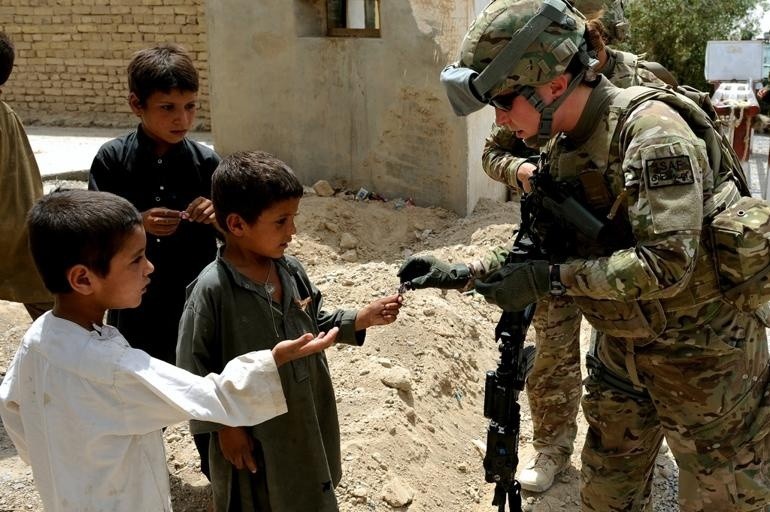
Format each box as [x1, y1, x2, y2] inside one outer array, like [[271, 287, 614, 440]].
[[519, 453, 571, 493]]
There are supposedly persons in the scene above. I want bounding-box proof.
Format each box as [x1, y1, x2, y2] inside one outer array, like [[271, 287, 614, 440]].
[[0, 28, 56, 322], [0, 186, 340, 512], [397, 1, 770, 511], [86, 43, 222, 482], [175, 149, 404, 512]]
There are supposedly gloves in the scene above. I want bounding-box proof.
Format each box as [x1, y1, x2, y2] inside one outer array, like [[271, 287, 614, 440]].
[[397, 256, 470, 290], [475, 260, 550, 312]]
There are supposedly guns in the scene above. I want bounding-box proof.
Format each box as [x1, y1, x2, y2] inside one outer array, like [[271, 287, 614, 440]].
[[485, 234, 554, 512]]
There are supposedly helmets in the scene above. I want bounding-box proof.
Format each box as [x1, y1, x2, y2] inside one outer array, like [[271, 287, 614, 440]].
[[460, 0, 586, 100]]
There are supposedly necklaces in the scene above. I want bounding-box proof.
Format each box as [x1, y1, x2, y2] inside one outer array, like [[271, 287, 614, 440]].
[[248, 259, 277, 294]]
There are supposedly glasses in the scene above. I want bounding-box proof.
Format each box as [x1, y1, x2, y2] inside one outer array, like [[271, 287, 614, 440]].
[[489, 91, 521, 112]]
